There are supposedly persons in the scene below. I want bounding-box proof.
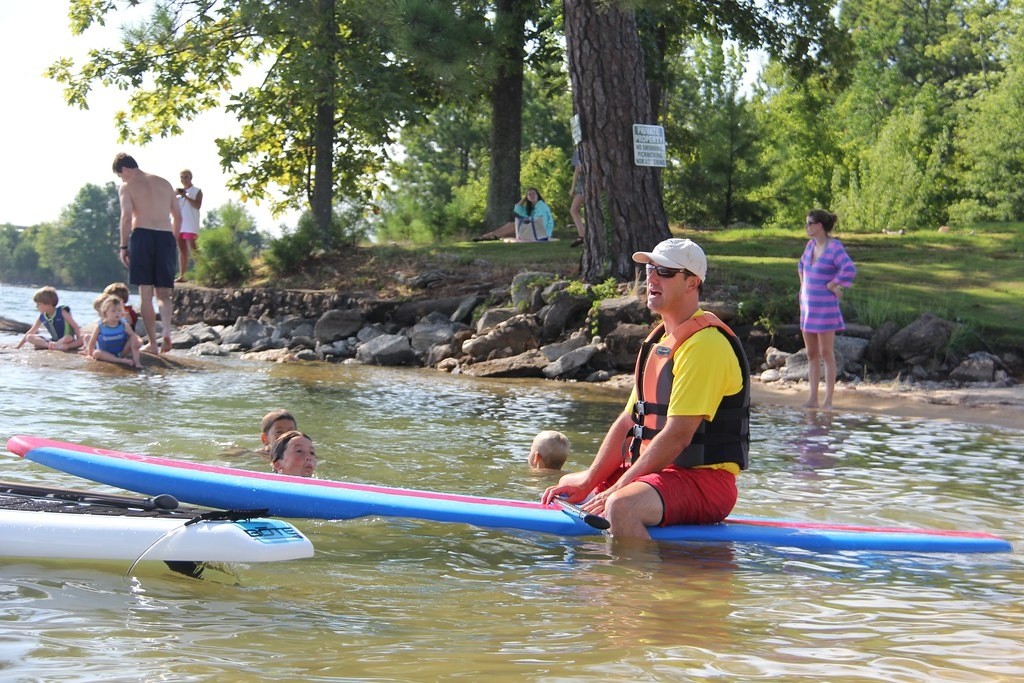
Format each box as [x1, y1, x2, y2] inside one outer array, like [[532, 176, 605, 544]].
[[568, 149, 585, 247], [174, 170, 203, 282], [528, 430, 569, 471], [473, 188, 553, 242], [13, 282, 143, 367], [261, 409, 316, 476], [542, 237, 750, 538], [798, 210, 855, 407], [112, 153, 182, 354]]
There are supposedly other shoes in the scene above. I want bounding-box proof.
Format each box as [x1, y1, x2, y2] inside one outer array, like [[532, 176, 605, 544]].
[[473, 234, 500, 242], [570, 236, 583, 248], [174, 278, 188, 282]]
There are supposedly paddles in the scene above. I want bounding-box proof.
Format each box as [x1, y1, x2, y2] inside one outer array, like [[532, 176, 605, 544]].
[[537, 490, 612, 532]]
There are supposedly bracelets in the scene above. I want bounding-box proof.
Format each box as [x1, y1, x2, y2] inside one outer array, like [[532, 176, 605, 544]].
[[120, 245, 128, 250]]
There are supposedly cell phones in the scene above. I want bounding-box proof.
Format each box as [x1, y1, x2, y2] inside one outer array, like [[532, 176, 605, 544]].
[[178, 189, 185, 193]]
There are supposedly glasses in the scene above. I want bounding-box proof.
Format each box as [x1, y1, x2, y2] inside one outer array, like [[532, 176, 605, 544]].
[[805, 221, 819, 226], [646, 263, 696, 278]]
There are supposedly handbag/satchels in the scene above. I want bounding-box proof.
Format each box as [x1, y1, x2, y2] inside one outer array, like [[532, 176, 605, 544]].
[[515, 216, 548, 242]]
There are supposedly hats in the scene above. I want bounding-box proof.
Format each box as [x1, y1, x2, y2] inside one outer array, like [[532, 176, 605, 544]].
[[632, 238, 707, 284]]
